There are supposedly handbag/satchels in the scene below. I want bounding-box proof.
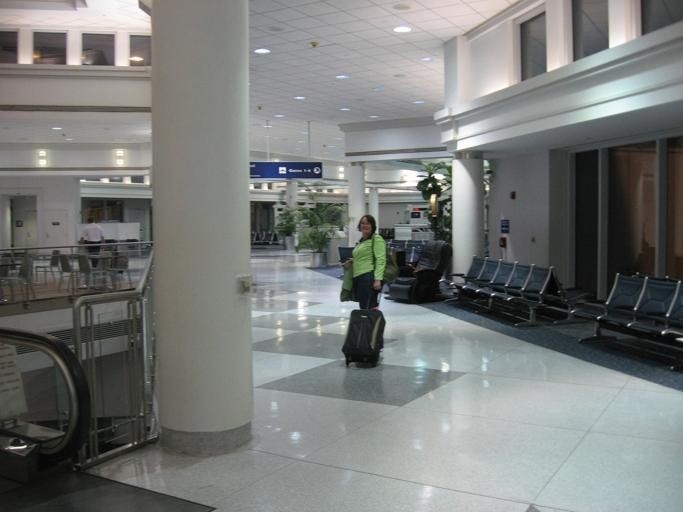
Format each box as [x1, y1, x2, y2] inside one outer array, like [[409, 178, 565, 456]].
[[383, 247, 400, 281]]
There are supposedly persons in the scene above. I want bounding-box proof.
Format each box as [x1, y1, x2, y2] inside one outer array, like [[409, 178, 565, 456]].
[[336, 214, 386, 314], [78, 216, 105, 268]]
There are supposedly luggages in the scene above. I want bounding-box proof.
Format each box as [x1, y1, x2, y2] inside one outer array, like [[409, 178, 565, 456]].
[[341, 288, 385, 368]]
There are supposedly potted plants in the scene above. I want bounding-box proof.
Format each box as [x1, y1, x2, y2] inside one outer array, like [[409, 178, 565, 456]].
[[287, 203, 346, 268]]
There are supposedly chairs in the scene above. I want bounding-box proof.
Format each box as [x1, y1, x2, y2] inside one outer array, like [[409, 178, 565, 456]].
[[251, 231, 279, 245], [387, 239, 452, 304], [570, 271, 683, 370], [439, 254, 569, 327], [0, 249, 132, 309], [338, 247, 354, 279]]
